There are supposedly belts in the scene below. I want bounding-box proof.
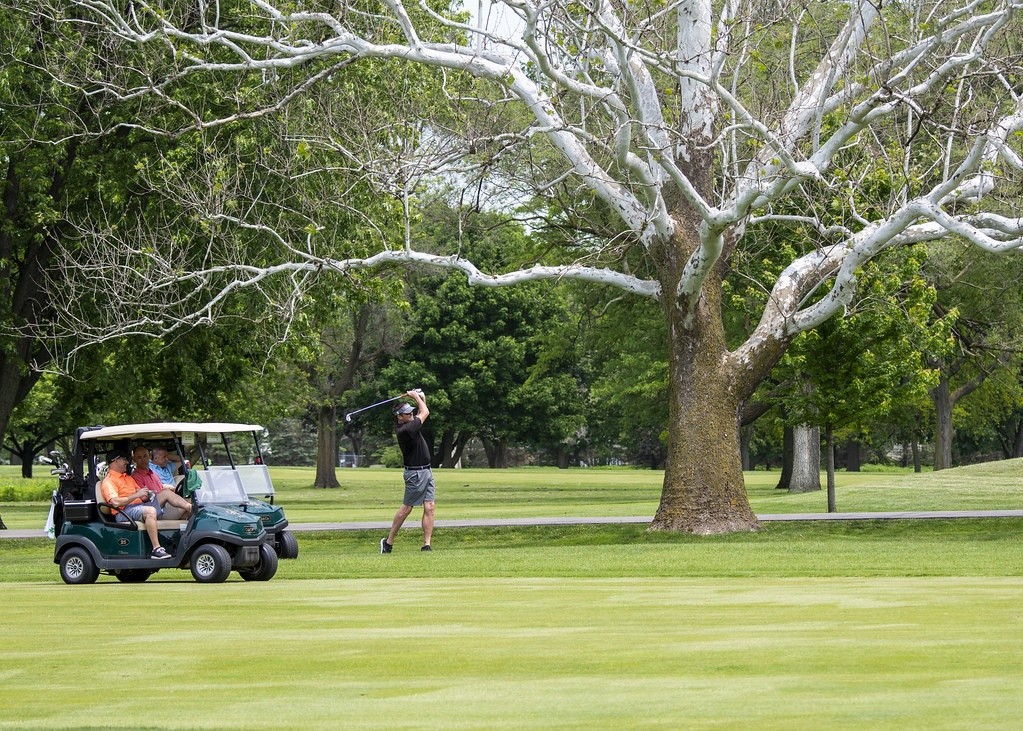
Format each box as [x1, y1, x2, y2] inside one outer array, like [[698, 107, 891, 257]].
[[404, 465, 432, 470]]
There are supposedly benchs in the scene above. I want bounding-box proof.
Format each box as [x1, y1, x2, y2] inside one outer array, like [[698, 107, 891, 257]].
[[93, 481, 189, 531]]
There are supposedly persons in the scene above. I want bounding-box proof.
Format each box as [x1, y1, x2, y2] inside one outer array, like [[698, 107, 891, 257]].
[[255, 456, 261, 465], [381, 389, 434, 553], [101, 446, 191, 560]]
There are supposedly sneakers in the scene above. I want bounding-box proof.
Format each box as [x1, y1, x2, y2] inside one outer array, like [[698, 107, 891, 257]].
[[151, 546, 172, 559], [190, 492, 207, 513], [421, 545, 431, 551], [381, 538, 392, 555]]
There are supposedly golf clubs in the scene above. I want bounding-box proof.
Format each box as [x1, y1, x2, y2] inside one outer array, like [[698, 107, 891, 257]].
[[38, 450, 107, 480], [345, 388, 423, 422]]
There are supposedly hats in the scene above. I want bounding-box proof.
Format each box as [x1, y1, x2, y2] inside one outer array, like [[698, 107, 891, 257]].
[[393, 403, 415, 416], [105, 449, 128, 464]]
